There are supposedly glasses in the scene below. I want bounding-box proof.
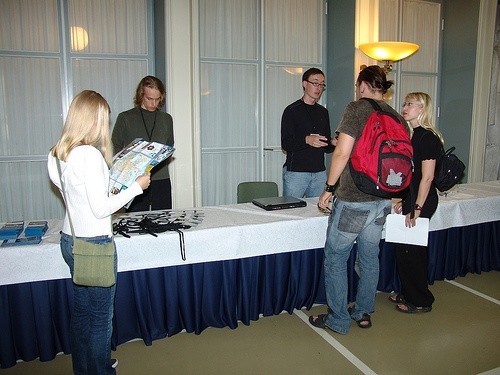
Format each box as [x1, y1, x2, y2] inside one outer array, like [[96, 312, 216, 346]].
[[304, 79, 326, 88]]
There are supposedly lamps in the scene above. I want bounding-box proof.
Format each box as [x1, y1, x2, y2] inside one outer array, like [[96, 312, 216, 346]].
[[358, 41, 420, 74]]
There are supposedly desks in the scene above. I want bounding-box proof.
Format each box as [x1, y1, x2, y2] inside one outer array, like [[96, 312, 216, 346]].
[[0, 175, 500, 375]]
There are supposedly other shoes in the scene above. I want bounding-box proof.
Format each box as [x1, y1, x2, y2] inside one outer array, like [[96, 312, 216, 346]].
[[111, 358, 119, 368]]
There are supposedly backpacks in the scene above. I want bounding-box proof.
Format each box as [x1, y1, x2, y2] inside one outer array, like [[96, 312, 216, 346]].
[[432, 146, 465, 192], [349, 97, 414, 199]]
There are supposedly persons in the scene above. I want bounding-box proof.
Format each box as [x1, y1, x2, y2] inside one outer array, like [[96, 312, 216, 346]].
[[48, 90, 150, 375], [309, 65, 410, 334], [281, 68, 339, 198], [112, 76, 174, 212], [389, 92, 445, 313]]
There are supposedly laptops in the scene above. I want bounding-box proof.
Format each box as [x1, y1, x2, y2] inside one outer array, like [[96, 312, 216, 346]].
[[251, 196, 307, 211]]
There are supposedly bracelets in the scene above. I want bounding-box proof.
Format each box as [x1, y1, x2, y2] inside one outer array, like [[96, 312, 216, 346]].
[[324, 181, 335, 192]]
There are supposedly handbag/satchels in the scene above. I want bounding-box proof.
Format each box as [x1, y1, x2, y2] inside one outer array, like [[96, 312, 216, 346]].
[[73, 237, 116, 288]]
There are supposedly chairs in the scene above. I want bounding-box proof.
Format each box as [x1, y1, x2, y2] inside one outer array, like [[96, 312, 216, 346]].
[[237, 182, 278, 203]]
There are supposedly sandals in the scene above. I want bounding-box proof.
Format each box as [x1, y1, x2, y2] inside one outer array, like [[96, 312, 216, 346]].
[[309, 314, 329, 328], [348, 305, 372, 328], [396, 302, 432, 314], [389, 294, 410, 303]]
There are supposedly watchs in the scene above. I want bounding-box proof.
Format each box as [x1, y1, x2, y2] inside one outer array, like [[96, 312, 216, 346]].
[[414, 204, 423, 211]]
